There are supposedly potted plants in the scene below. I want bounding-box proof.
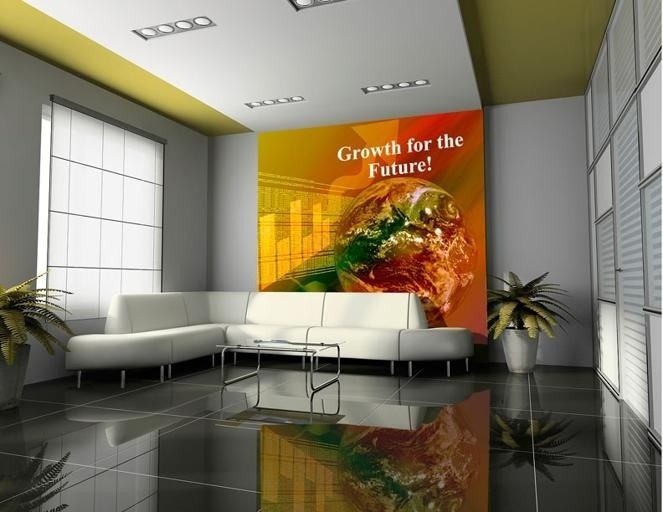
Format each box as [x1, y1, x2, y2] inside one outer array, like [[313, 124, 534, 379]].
[[0, 405, 73, 512], [0, 269, 77, 411], [481, 271, 584, 373]]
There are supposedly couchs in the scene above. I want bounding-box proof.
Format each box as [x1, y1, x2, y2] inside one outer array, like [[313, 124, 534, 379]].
[[63, 374, 474, 449], [65, 290, 475, 391], [490, 375, 584, 483]]
